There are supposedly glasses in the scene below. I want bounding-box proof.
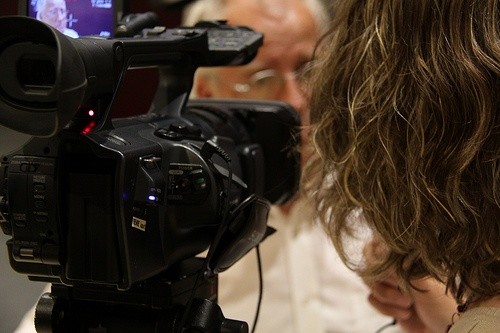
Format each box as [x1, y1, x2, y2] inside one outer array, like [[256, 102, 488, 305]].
[[207, 61, 313, 100]]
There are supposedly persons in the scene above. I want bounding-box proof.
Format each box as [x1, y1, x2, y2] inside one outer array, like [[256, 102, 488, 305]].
[[35, 0, 79, 39], [14, 0, 500, 333]]
